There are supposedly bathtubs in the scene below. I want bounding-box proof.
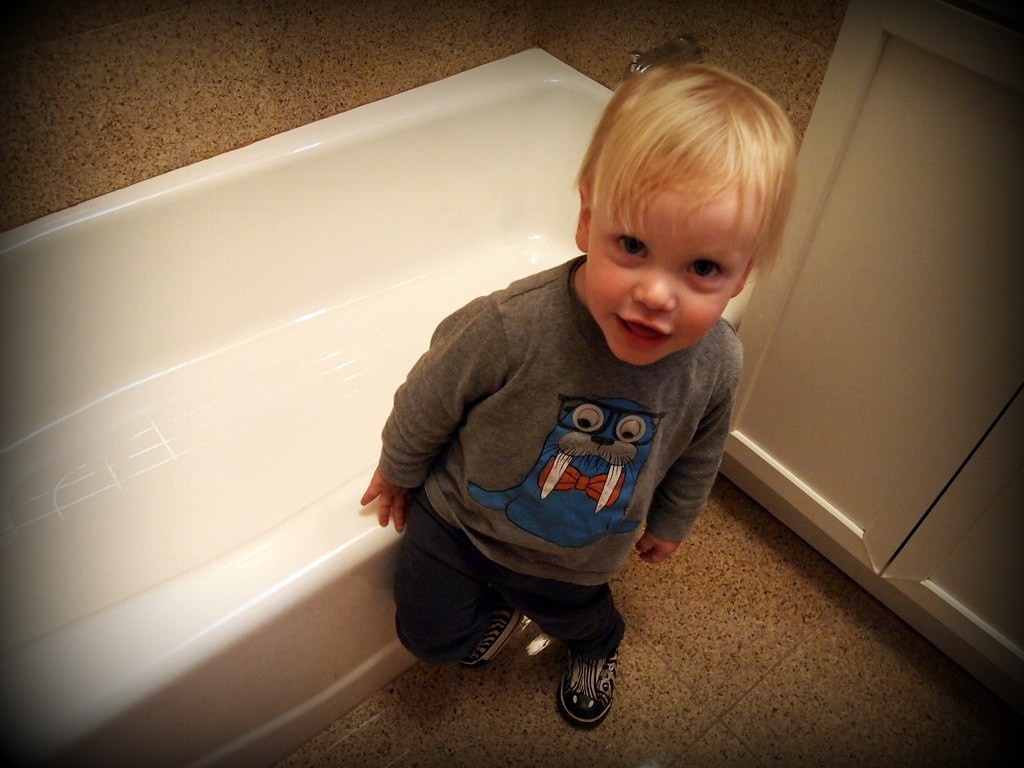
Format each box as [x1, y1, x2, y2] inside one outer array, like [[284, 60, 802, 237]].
[[1, 42, 766, 768]]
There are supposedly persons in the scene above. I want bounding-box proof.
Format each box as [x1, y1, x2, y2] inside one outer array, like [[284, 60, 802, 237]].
[[359, 63, 801, 729]]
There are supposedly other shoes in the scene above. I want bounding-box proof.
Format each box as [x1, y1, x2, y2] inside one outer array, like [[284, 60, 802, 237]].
[[557, 649, 618, 730], [460, 602, 521, 667]]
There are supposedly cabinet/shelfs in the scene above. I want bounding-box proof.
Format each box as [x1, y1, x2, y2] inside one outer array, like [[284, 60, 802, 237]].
[[718, 1, 1023, 718]]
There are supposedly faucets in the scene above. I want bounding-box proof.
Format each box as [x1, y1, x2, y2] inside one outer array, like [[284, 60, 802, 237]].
[[620, 33, 706, 85]]
